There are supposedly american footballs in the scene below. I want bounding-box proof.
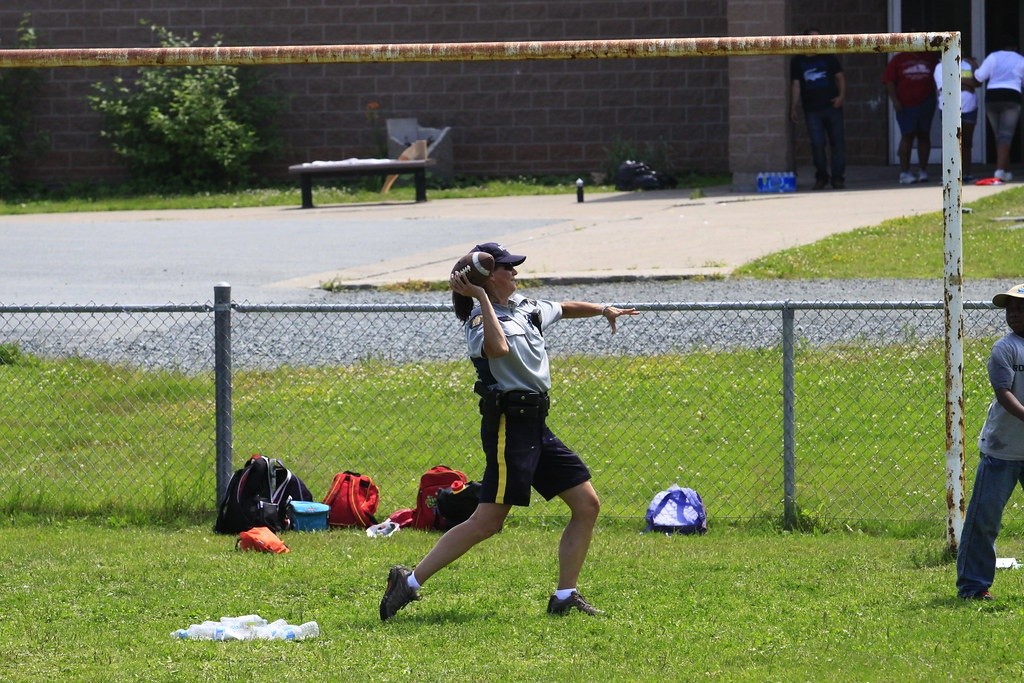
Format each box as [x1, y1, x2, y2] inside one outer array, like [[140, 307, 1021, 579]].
[[451, 251, 495, 287]]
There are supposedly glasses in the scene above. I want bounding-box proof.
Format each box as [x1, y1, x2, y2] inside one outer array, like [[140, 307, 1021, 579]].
[[497, 262, 514, 271]]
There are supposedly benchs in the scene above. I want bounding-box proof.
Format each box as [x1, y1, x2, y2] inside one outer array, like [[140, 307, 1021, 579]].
[[289, 157, 437, 208]]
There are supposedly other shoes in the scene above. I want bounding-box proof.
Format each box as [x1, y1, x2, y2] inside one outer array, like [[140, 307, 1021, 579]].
[[832, 181, 846, 190], [918, 169, 928, 183], [811, 178, 829, 189], [994, 169, 1012, 182], [963, 592, 993, 601], [899, 171, 918, 184]]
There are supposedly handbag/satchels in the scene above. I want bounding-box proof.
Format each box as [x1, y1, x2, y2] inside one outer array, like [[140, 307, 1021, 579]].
[[237, 526, 287, 557], [217, 455, 313, 531], [433, 480, 483, 531]]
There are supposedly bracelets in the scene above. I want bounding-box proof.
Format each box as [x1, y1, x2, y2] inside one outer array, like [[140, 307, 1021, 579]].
[[602, 303, 610, 315]]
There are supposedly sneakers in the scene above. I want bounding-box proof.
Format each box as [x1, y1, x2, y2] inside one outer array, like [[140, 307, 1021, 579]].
[[380, 565, 423, 620], [547, 591, 605, 617]]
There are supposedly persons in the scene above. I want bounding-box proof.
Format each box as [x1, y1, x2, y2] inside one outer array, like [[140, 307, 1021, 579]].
[[956, 284, 1024, 602], [379, 243, 639, 623], [933, 23, 983, 180], [881, 28, 937, 184], [965, 32, 1024, 181], [790, 28, 847, 189]]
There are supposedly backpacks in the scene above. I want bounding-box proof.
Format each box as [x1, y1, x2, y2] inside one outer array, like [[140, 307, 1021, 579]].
[[645, 483, 706, 533], [323, 470, 379, 528], [414, 465, 466, 528]]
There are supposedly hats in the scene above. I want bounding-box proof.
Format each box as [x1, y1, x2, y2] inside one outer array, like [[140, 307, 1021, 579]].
[[469, 243, 526, 266], [993, 284, 1024, 307]]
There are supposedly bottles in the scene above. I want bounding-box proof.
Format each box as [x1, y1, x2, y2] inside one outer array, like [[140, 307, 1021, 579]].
[[576, 178, 583, 202], [764, 172, 770, 192], [790, 172, 796, 190], [758, 172, 764, 192], [170, 615, 319, 641], [770, 172, 776, 192], [784, 172, 790, 192], [776, 172, 784, 192], [451, 481, 466, 495]]
